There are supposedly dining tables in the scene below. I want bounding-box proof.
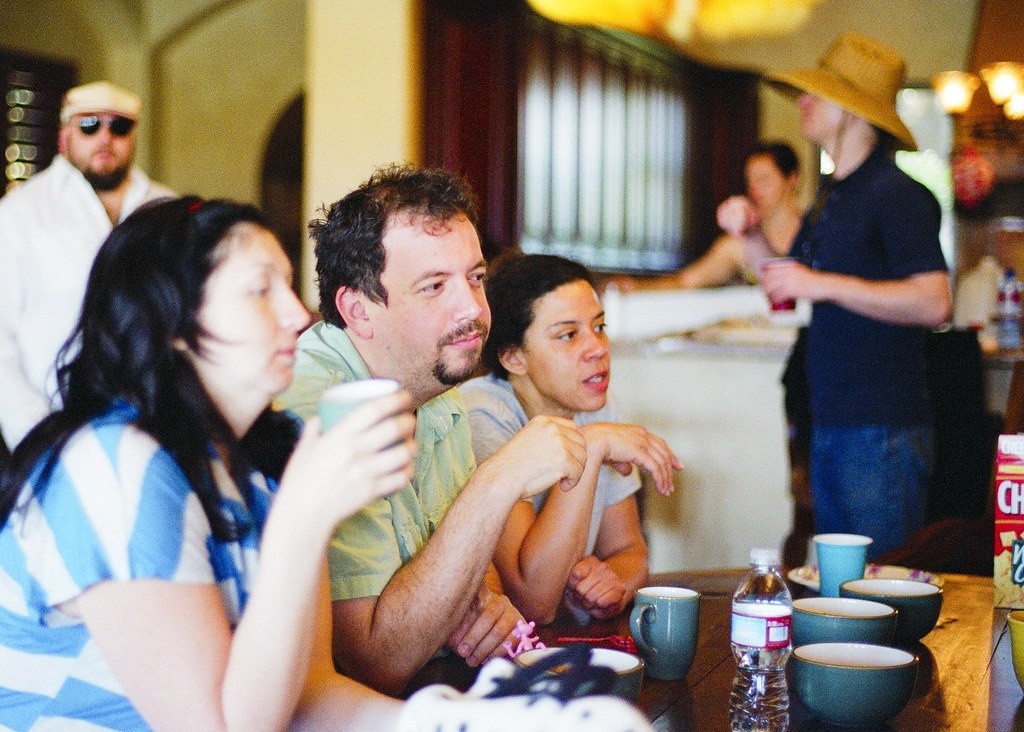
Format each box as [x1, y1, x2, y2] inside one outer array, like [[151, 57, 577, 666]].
[[392, 560, 998, 732]]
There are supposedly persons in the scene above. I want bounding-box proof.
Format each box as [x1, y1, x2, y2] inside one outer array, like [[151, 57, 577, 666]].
[[459, 248, 681, 630], [610, 38, 952, 564], [272, 165, 588, 699], [0, 195, 417, 732], [0, 80, 183, 455]]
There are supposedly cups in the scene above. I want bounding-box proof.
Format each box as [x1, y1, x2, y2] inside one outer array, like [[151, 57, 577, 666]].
[[320, 378, 401, 432], [812, 533, 873, 598], [630, 585, 703, 681], [758, 256, 798, 311]]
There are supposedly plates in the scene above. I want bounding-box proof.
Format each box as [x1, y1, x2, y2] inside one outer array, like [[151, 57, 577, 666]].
[[788, 564, 945, 594]]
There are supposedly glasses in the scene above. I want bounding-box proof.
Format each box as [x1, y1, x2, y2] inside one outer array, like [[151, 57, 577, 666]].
[[68, 115, 135, 137]]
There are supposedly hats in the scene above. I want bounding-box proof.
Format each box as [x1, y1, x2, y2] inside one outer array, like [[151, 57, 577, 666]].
[[59, 82, 141, 126], [763, 33, 918, 151]]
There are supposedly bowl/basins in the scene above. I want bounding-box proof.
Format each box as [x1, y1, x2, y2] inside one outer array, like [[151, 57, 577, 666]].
[[838, 578, 944, 641], [791, 641, 919, 728], [1006, 610, 1024, 695], [516, 645, 644, 709], [790, 598, 899, 647]]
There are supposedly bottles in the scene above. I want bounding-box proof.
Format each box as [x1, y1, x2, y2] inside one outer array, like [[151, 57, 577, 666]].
[[997, 267, 1023, 350], [728, 547, 793, 732]]
